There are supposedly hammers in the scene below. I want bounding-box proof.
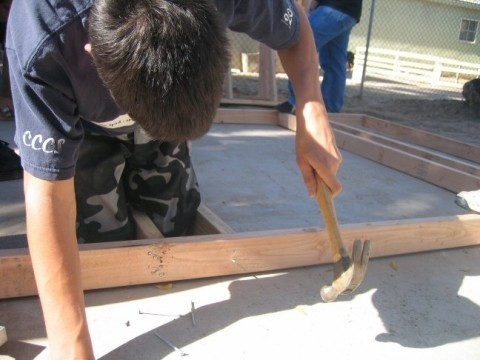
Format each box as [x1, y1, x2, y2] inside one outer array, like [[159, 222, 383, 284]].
[[312, 169, 371, 302]]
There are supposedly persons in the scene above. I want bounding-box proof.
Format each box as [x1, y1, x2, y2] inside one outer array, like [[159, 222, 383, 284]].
[[276, 0, 362, 115], [4, 0, 342, 360]]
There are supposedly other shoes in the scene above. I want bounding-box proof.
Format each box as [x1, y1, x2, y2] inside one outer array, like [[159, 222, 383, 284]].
[[274, 101, 295, 114]]
